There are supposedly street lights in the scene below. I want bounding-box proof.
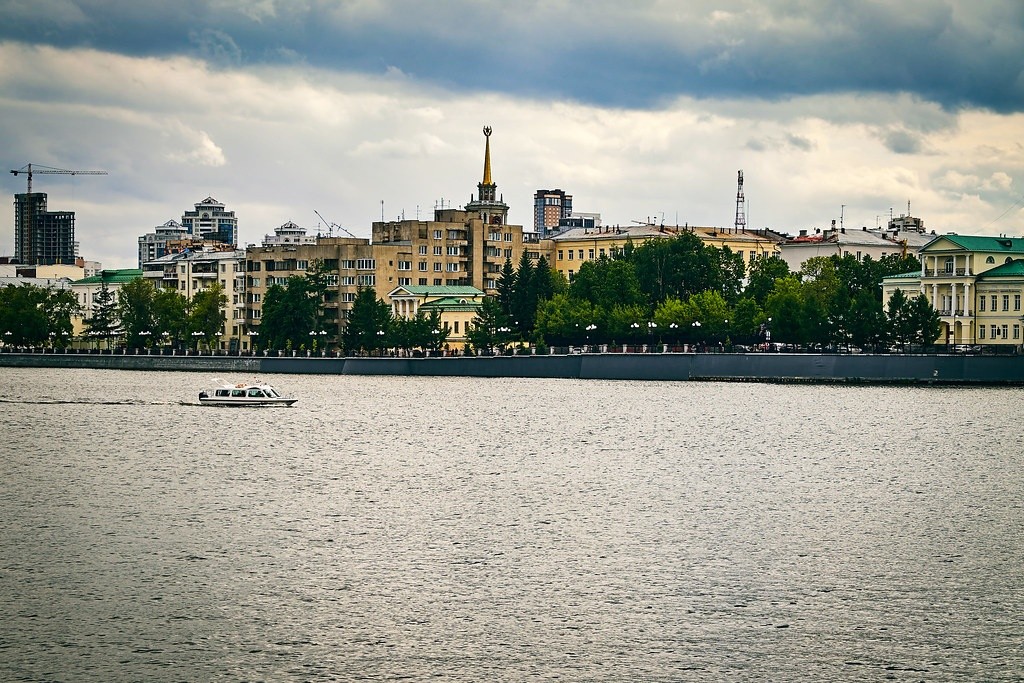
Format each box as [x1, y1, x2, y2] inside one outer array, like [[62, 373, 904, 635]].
[[498, 327, 511, 356], [215, 331, 222, 354], [586, 324, 597, 352], [319, 329, 327, 347], [648, 322, 658, 353], [309, 331, 317, 356], [692, 321, 702, 354], [670, 323, 679, 352], [631, 322, 640, 353], [191, 331, 205, 355], [377, 330, 385, 356], [162, 331, 169, 355], [953, 312, 963, 352], [247, 331, 259, 351]]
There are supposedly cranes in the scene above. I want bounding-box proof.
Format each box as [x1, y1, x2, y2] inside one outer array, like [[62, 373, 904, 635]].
[[9, 162, 110, 192]]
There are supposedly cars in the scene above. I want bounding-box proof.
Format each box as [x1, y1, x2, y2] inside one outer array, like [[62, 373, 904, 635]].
[[971, 345, 993, 354], [952, 344, 972, 353], [731, 342, 863, 354]]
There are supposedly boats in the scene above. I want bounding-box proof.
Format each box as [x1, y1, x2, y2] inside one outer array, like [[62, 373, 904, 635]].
[[199, 378, 299, 408]]
